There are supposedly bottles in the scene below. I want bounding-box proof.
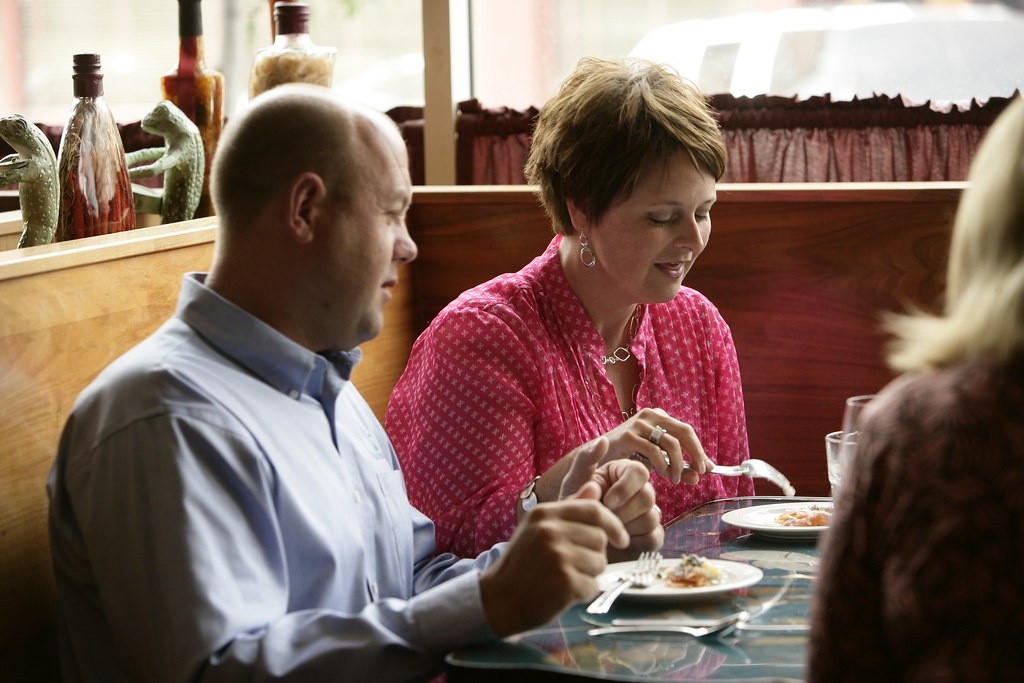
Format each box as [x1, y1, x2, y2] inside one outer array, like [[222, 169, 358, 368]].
[[57, 53, 136, 242], [161, 0, 225, 219], [248, 1, 337, 100]]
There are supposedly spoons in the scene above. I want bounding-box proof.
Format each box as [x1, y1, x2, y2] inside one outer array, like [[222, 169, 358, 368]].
[[587, 611, 750, 638]]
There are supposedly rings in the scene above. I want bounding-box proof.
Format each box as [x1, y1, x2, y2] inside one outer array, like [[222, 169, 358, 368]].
[[649, 425, 667, 444], [653, 505, 662, 521]]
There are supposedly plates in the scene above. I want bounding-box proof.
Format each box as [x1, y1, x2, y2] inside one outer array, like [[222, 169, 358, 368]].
[[721, 501, 834, 540], [594, 558, 764, 604]]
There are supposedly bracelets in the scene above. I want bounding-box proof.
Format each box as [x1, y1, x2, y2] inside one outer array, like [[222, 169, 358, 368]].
[[520, 476, 540, 513]]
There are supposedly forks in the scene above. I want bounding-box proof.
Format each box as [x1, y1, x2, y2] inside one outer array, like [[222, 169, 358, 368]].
[[664, 457, 791, 491], [585, 551, 663, 614]]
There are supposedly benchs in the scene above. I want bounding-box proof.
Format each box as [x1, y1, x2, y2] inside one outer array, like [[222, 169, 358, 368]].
[[1, 181, 1024, 683]]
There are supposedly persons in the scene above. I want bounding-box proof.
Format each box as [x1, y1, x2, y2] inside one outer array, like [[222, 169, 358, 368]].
[[45, 86, 666, 683], [806, 99, 1024, 683], [385, 58, 755, 560], [525, 500, 790, 681]]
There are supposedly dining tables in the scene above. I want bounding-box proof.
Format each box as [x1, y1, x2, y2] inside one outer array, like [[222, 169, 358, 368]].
[[445, 496, 836, 683]]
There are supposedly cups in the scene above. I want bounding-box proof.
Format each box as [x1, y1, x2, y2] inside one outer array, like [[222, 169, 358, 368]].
[[836, 394, 877, 468], [825, 430, 846, 486]]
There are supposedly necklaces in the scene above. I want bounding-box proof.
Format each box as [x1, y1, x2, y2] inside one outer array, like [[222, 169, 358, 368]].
[[602, 304, 642, 420]]
